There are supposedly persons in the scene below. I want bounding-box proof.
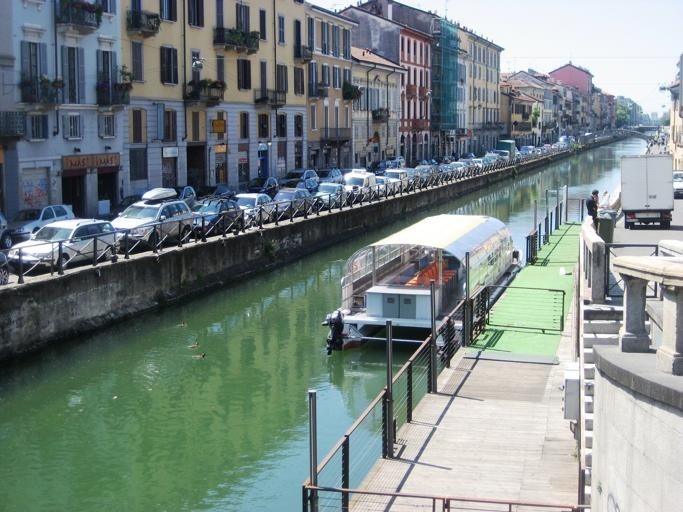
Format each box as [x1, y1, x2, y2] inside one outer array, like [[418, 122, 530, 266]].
[[585, 188, 600, 233]]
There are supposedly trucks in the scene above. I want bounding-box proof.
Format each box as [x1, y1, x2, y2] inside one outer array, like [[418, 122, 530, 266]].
[[619, 152, 674, 229], [494, 140, 516, 163]]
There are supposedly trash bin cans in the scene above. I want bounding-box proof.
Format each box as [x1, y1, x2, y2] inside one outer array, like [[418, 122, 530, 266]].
[[594, 209, 618, 243]]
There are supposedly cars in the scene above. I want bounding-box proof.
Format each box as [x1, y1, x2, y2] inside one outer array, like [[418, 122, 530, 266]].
[[0, 203, 120, 284], [273, 157, 408, 219], [406, 153, 503, 191], [520, 136, 575, 156], [673, 171, 683, 199], [105, 177, 279, 253]]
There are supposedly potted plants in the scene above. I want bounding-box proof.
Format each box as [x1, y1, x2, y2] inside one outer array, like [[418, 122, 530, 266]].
[[117, 64, 133, 89], [53, 79, 66, 87]]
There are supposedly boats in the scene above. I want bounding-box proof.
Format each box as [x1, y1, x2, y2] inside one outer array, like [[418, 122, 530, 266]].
[[322, 214, 515, 356]]
